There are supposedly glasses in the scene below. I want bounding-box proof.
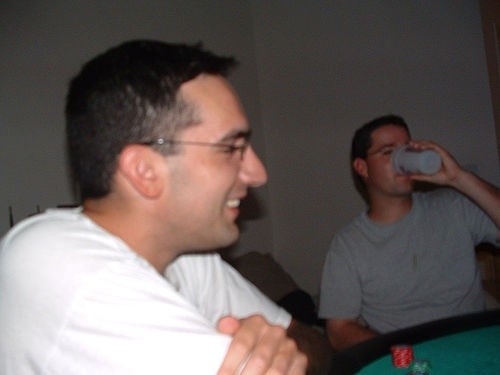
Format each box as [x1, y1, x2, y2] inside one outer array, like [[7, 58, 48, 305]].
[[138, 139, 252, 163]]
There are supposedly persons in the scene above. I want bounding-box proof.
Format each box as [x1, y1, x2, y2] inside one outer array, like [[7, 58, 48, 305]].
[[0, 38, 339, 375], [316, 114, 499, 352]]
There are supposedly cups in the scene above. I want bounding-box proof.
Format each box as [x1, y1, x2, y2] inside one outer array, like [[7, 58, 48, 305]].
[[391, 144, 441, 176]]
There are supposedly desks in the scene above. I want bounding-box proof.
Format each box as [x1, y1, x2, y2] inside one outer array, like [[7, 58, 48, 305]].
[[305, 310, 500, 375]]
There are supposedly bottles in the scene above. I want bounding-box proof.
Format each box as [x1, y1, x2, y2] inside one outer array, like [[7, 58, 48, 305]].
[[406, 358, 432, 375]]
